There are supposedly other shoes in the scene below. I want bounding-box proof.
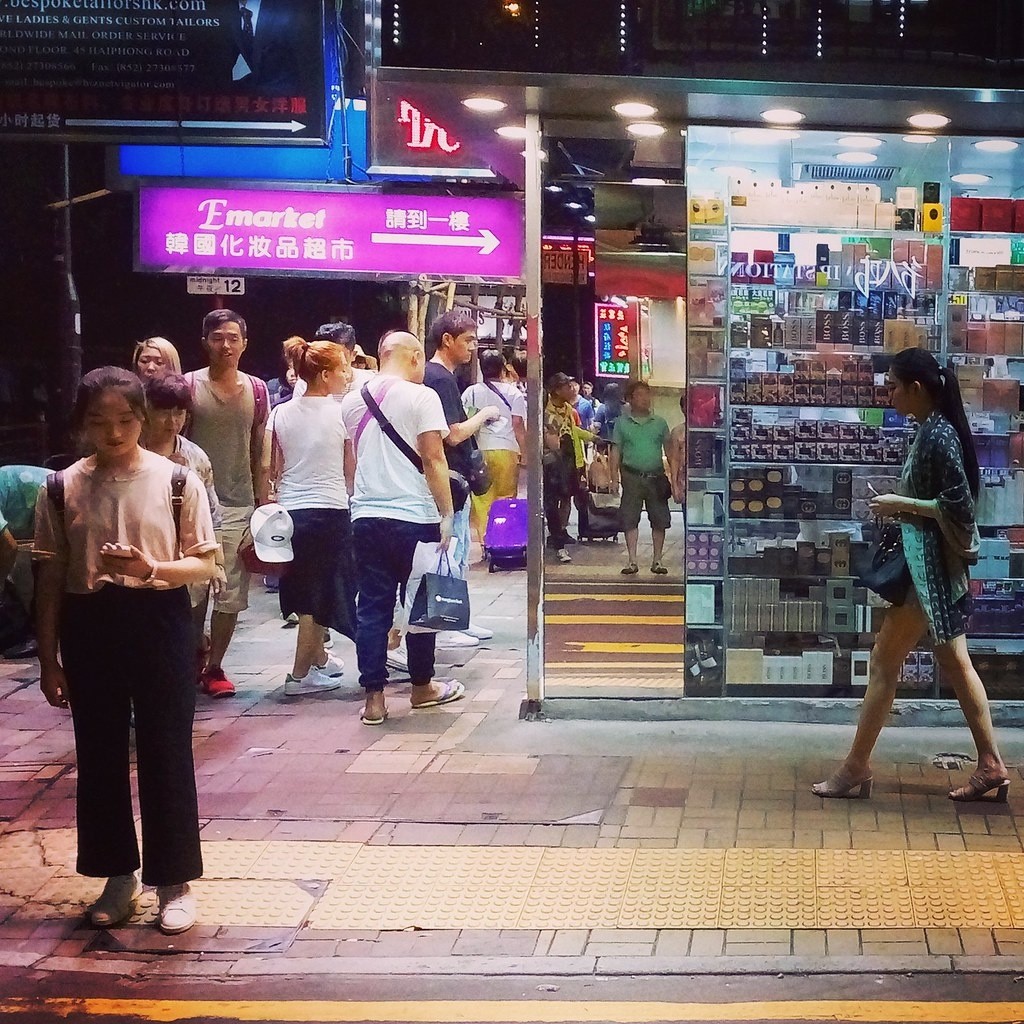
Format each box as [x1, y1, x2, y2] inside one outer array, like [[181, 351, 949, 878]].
[[561, 528, 576, 544], [4, 636, 42, 661]]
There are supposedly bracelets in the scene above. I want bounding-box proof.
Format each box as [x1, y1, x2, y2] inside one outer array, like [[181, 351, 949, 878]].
[[912, 499, 917, 516], [141, 561, 157, 584]]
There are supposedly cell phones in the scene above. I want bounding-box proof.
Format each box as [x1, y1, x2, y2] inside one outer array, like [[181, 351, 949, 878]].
[[102, 542, 133, 558]]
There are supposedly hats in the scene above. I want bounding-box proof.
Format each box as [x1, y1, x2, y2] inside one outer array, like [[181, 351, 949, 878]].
[[543, 371, 574, 393], [249, 503, 295, 563], [351, 343, 379, 370]]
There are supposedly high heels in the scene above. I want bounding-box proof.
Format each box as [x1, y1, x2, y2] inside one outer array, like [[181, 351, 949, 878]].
[[947, 776, 1011, 803], [810, 768, 875, 800]]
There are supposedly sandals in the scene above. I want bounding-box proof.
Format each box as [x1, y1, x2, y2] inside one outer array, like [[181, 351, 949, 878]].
[[620, 561, 639, 574], [649, 560, 669, 574]]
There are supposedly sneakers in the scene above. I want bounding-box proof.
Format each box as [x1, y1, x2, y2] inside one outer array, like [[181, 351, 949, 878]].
[[157, 883, 197, 934], [556, 548, 573, 562], [90, 872, 145, 927], [198, 613, 494, 727]]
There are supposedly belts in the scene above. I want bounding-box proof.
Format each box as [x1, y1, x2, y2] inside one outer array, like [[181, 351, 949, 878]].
[[627, 466, 665, 478]]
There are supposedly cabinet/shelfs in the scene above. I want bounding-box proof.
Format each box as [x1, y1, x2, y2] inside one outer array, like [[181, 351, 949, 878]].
[[682, 124, 1024, 699]]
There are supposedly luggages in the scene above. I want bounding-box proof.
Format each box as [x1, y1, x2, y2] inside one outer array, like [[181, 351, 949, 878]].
[[578, 436, 624, 539], [480, 492, 535, 573]]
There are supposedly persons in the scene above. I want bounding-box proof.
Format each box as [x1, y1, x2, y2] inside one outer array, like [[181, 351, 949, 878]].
[[35, 366, 221, 935], [134, 308, 526, 724], [130, 372, 229, 726], [0, 464, 57, 659], [541, 372, 687, 575], [810, 348, 1012, 801]]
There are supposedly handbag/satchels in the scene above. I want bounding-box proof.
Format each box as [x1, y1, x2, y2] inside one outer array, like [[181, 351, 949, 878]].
[[433, 448, 493, 514], [402, 536, 471, 637], [585, 442, 617, 493], [655, 474, 672, 499], [457, 385, 481, 440], [239, 539, 292, 578], [853, 521, 913, 609]]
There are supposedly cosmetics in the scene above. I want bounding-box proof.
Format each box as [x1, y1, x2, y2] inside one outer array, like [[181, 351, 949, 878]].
[[687, 167, 1024, 685]]
